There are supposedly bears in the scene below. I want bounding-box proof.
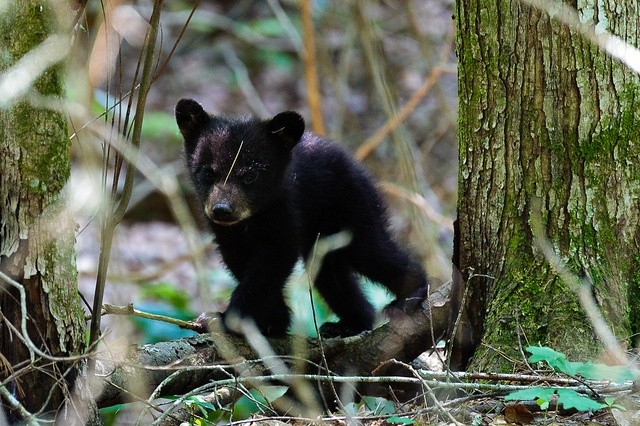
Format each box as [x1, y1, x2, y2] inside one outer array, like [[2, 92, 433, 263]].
[[174, 97, 431, 339]]
